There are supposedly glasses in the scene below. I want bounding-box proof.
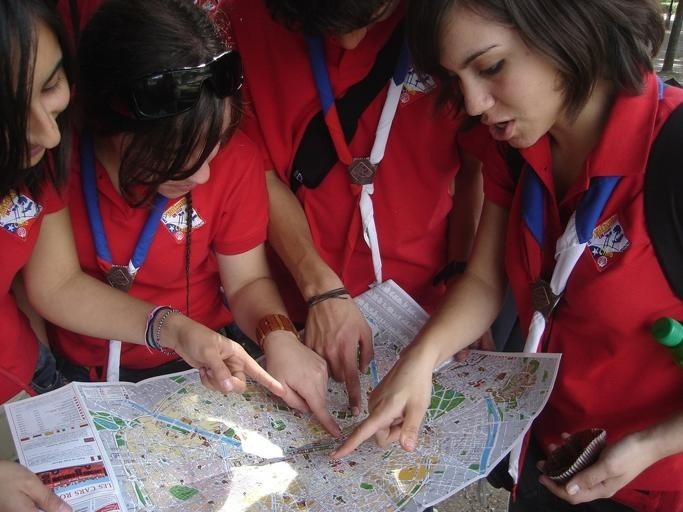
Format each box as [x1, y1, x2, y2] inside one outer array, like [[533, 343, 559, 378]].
[[124, 50, 243, 119]]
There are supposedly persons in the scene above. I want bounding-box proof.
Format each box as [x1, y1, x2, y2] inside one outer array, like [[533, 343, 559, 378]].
[[1, 0, 289, 512], [16, 0, 344, 439], [211, 1, 496, 418], [331, 0, 682, 512]]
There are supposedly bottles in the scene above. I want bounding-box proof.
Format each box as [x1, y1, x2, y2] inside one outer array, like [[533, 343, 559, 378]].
[[651, 317, 683, 367]]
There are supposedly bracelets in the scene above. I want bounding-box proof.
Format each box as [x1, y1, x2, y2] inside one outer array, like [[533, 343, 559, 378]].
[[256, 314, 301, 354], [144, 304, 180, 357], [307, 288, 349, 307]]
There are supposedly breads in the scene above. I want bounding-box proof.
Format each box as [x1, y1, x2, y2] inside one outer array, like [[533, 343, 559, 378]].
[[543, 427, 607, 485]]
